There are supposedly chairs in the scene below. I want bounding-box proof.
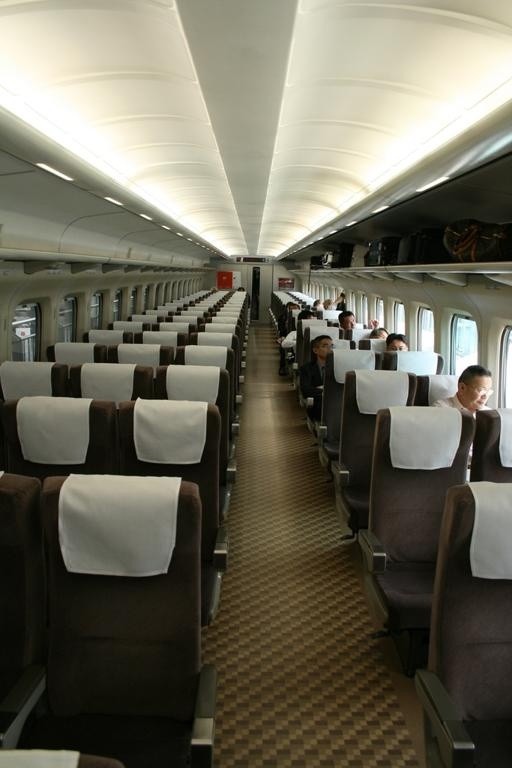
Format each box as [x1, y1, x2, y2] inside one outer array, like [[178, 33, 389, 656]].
[[135, 331, 185, 345], [190, 332, 246, 373], [82, 329, 133, 345], [345, 328, 372, 348], [157, 364, 238, 488], [107, 345, 174, 365], [145, 289, 251, 316], [471, 408, 512, 483], [416, 374, 462, 406], [287, 320, 328, 363], [0, 475, 217, 768], [165, 315, 203, 327], [287, 290, 344, 319], [206, 316, 247, 349], [152, 323, 196, 340], [334, 322, 366, 339], [358, 406, 474, 680], [1, 362, 69, 397], [1, 397, 118, 476], [292, 327, 343, 391], [333, 370, 417, 545], [108, 320, 150, 333], [269, 290, 287, 336], [384, 350, 445, 376], [314, 349, 383, 468], [46, 341, 106, 366], [120, 398, 227, 627], [300, 338, 356, 433], [199, 323, 246, 358], [360, 338, 388, 370], [1, 474, 39, 699], [128, 314, 164, 325], [176, 345, 242, 410], [72, 363, 153, 401], [413, 481, 512, 767]]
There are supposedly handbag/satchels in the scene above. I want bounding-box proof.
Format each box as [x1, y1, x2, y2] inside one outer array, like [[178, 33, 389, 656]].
[[441, 217, 512, 262]]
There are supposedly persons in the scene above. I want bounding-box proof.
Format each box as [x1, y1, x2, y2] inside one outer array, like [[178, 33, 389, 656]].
[[370, 328, 389, 340], [282, 310, 314, 362], [278, 302, 295, 335], [387, 333, 409, 351], [238, 287, 250, 308], [286, 304, 300, 331], [300, 335, 334, 421], [339, 311, 356, 329], [430, 365, 493, 412], [323, 300, 335, 310]]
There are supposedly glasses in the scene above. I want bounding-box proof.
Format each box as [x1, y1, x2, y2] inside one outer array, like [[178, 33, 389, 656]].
[[314, 345, 337, 349], [388, 346, 409, 349], [466, 381, 494, 399]]
